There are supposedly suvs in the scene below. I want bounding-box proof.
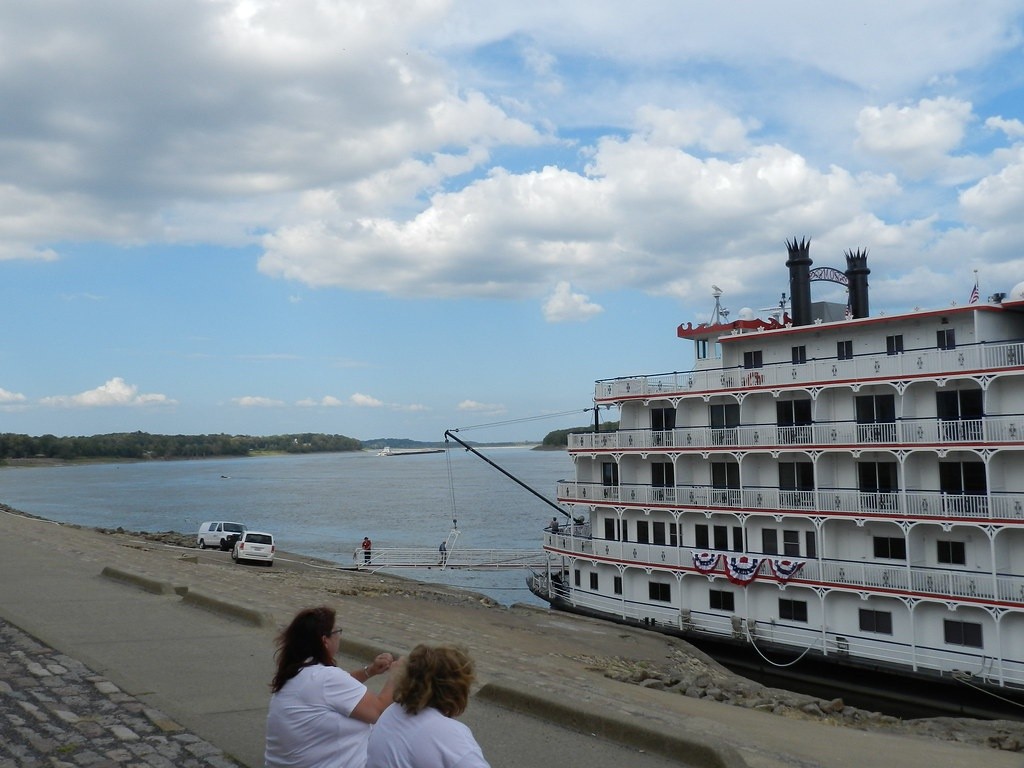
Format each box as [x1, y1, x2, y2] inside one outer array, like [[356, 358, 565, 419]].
[[231, 530, 276, 567]]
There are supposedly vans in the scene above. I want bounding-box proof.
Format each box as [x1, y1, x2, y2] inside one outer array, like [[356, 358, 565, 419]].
[[197, 521, 249, 550]]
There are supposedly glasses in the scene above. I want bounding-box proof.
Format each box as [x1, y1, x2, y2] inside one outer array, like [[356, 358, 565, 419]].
[[325, 627, 342, 638]]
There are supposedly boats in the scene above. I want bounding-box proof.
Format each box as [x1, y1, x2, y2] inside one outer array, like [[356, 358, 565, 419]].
[[437, 232, 1024, 730]]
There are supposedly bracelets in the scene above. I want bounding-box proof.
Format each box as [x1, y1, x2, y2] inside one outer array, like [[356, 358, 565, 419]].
[[364, 665, 370, 678]]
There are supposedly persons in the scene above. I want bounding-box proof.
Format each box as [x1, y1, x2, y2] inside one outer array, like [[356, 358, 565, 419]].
[[367, 641, 490, 768], [439, 541, 447, 564], [362, 537, 372, 565], [265, 606, 406, 768], [550, 517, 559, 534]]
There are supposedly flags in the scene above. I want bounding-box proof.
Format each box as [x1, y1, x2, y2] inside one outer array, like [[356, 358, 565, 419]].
[[969, 282, 979, 303]]
